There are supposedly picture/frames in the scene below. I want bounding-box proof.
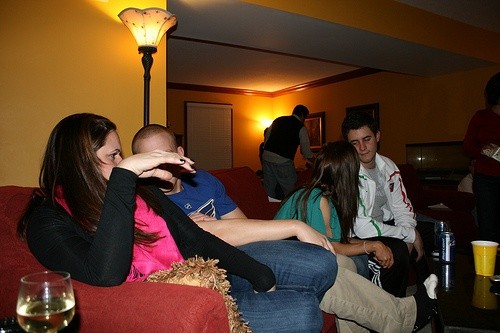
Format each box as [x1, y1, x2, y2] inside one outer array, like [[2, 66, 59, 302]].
[[345, 102, 380, 150], [303, 111, 325, 152]]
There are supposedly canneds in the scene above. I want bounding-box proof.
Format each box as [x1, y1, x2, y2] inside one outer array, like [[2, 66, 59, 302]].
[[440, 263, 456, 291], [439, 232, 456, 263]]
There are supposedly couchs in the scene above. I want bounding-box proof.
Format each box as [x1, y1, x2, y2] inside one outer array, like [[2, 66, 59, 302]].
[[298, 164, 479, 285], [1, 166, 338, 333]]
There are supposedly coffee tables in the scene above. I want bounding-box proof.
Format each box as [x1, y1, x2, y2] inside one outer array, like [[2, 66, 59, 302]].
[[418, 247, 500, 333]]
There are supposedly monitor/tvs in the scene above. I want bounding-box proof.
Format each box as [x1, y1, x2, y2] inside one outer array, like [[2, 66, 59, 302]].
[[407, 142, 473, 184]]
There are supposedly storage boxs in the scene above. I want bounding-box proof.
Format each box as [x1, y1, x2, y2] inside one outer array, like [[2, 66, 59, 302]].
[[405, 141, 470, 186]]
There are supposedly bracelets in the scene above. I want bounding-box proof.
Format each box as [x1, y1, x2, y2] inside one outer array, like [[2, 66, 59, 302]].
[[364, 241, 370, 254]]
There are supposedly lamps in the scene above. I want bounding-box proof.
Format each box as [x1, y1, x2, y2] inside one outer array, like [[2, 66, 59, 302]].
[[117, 7, 177, 126]]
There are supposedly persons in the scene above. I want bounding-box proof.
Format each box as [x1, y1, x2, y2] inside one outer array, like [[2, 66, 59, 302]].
[[259, 105, 316, 200], [131, 124, 434, 333], [273, 140, 394, 281], [17, 113, 338, 333], [462, 72, 500, 244], [341, 113, 438, 297]]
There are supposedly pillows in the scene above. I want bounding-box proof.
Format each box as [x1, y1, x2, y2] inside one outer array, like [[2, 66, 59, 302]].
[[142, 255, 252, 333]]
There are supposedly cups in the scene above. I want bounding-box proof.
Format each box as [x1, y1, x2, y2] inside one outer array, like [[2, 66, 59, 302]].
[[471, 241, 500, 276], [16, 270, 75, 333]]
